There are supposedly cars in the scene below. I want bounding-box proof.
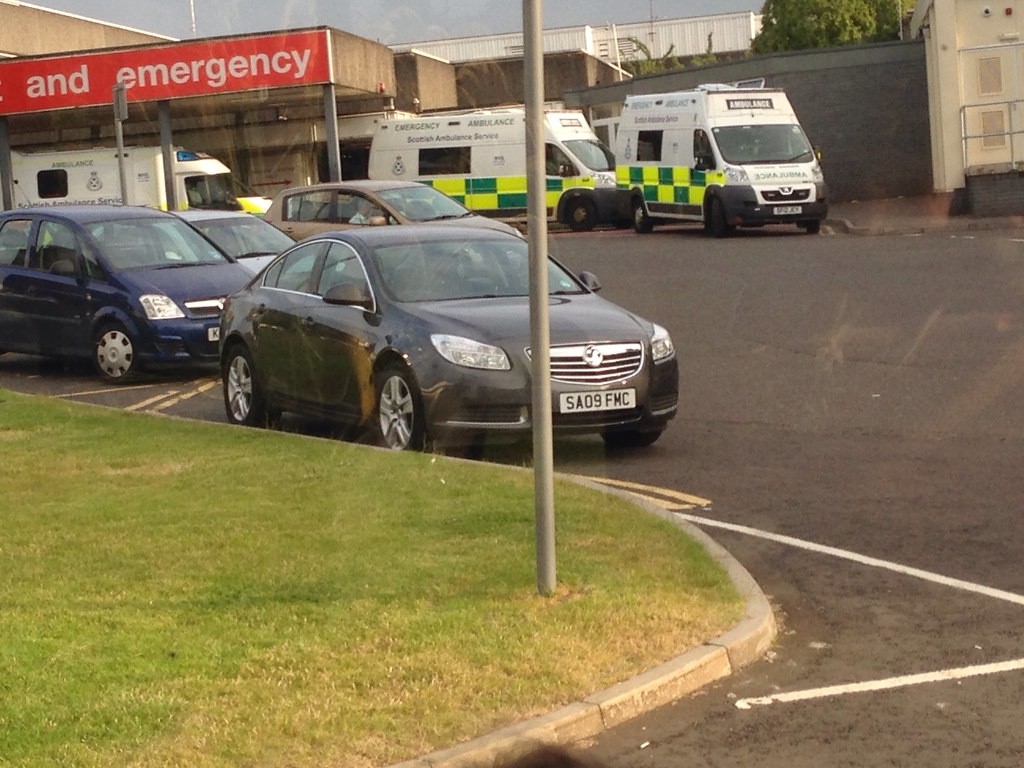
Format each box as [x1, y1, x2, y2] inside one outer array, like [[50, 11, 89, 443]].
[[218, 225, 679, 451], [89, 209, 299, 284], [265, 178, 524, 263], [0, 207, 256, 389]]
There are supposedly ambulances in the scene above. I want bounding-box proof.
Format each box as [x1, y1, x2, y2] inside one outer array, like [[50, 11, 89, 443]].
[[365, 110, 636, 231], [614, 86, 830, 235]]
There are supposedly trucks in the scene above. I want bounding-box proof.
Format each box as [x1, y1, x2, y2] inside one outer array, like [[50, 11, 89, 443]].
[[10, 145, 275, 209]]
